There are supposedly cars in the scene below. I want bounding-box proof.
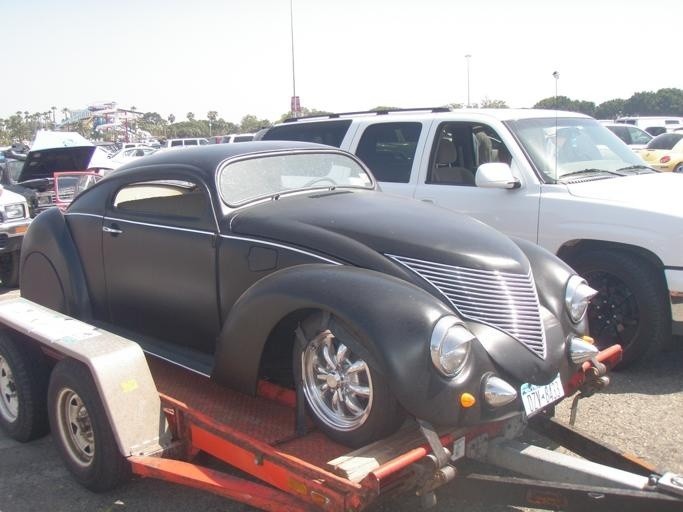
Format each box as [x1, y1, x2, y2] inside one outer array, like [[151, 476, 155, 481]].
[[18, 140, 598, 451], [0, 117, 254, 284], [530, 115, 683, 174]]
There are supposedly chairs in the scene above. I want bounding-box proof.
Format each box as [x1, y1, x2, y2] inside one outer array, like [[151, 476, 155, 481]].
[[430, 139, 475, 185]]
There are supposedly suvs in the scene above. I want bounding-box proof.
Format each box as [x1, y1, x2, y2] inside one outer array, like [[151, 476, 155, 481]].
[[0, 183, 33, 288], [251, 106, 682, 373]]
